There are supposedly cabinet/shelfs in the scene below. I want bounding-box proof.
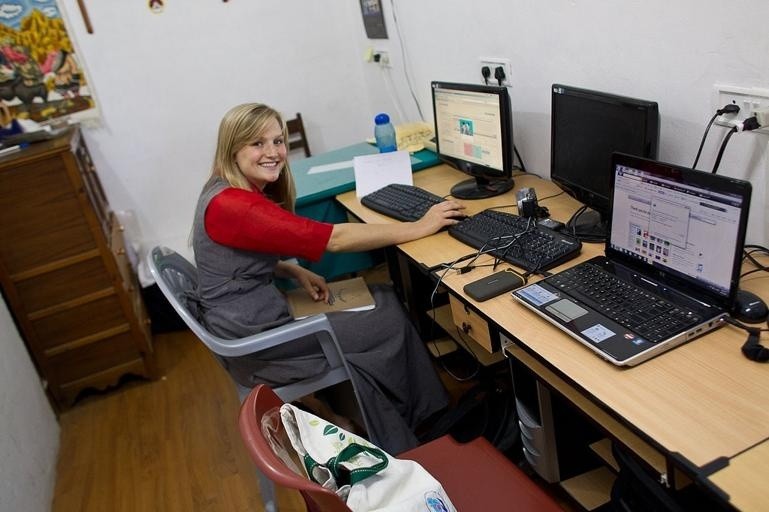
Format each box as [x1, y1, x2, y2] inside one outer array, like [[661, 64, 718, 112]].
[[0, 120, 161, 419]]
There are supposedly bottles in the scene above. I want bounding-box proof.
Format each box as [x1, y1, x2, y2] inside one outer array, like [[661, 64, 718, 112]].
[[374, 114, 398, 153]]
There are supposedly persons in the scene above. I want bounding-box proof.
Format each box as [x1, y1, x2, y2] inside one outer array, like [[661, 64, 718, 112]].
[[175, 103, 467, 456]]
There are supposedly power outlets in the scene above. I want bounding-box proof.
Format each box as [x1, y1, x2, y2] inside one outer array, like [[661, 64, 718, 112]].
[[370, 51, 391, 67], [477, 59, 512, 87], [713, 85, 768, 132]]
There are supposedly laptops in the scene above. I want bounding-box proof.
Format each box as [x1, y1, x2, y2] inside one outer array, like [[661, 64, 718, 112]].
[[511, 152, 752, 370]]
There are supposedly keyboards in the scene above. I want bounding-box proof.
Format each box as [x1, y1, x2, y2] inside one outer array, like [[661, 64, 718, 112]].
[[361, 184, 446, 222], [450, 209, 582, 275]]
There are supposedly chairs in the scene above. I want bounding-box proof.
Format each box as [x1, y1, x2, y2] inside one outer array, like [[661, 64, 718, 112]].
[[236, 385, 566, 512], [285, 112, 311, 159], [144, 244, 378, 451]]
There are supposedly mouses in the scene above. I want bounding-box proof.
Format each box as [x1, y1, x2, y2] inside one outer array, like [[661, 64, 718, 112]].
[[437, 209, 469, 233], [736, 287, 769, 324]]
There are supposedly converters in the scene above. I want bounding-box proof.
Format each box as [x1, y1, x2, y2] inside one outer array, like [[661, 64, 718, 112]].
[[539, 218, 565, 232], [522, 199, 537, 219]]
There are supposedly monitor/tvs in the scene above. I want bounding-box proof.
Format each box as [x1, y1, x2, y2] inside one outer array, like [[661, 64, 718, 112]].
[[550, 84, 660, 243], [431, 81, 515, 201]]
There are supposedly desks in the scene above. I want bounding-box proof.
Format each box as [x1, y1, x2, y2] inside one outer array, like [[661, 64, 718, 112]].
[[697, 440, 767, 512], [425, 234, 768, 512], [275, 141, 445, 289], [335, 161, 593, 337]]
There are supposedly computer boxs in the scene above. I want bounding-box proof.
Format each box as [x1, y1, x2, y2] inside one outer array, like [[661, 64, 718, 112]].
[[509, 364, 609, 482]]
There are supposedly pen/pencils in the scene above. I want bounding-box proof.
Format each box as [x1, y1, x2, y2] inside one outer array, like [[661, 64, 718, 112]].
[[0, 141, 27, 155]]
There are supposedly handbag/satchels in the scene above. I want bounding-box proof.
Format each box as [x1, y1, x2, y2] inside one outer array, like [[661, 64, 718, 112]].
[[281, 405, 456, 512]]
